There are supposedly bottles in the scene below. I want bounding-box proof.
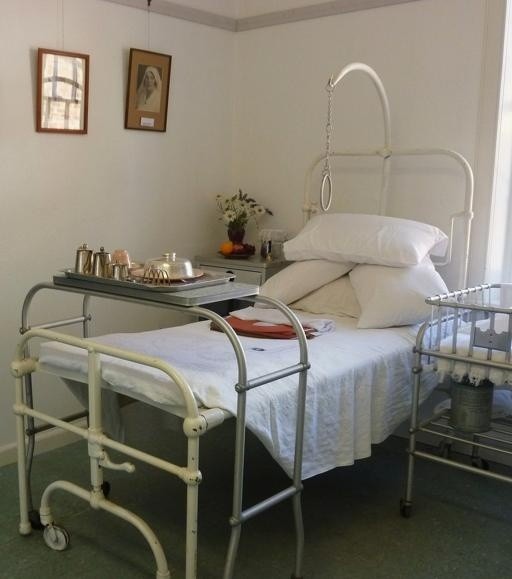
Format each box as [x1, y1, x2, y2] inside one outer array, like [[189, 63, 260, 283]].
[[75, 243, 129, 282]]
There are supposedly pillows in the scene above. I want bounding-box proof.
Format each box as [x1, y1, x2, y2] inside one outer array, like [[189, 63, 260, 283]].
[[257, 211, 451, 331]]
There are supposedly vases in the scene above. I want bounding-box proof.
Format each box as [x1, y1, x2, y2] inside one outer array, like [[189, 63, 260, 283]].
[[227, 228, 246, 243]]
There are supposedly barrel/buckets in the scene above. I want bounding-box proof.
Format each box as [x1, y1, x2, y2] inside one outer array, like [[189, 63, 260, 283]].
[[448, 379, 494, 432]]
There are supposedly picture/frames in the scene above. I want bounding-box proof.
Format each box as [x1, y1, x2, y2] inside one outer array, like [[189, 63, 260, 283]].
[[125, 46, 173, 133], [36, 49, 92, 135]]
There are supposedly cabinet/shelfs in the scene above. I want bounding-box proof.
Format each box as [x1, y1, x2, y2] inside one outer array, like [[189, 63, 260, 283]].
[[397, 283, 511, 518]]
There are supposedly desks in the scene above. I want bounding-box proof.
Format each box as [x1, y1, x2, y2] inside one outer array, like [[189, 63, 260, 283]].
[[22, 261, 311, 578]]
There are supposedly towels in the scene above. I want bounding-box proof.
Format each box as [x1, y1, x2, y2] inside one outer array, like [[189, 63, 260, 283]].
[[209, 302, 335, 341]]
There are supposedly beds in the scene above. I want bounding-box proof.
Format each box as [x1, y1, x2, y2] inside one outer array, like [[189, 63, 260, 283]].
[[0, 148, 477, 578]]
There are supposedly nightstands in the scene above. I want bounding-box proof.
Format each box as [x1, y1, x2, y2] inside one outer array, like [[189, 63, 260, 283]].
[[193, 253, 292, 324]]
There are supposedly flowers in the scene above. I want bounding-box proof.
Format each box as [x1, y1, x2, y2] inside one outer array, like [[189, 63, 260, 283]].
[[214, 187, 274, 233]]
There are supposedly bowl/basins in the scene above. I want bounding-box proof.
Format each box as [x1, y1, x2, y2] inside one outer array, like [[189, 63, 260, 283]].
[[217, 250, 256, 259]]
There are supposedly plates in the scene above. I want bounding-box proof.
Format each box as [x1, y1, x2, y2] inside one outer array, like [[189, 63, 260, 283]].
[[132, 267, 203, 280]]
